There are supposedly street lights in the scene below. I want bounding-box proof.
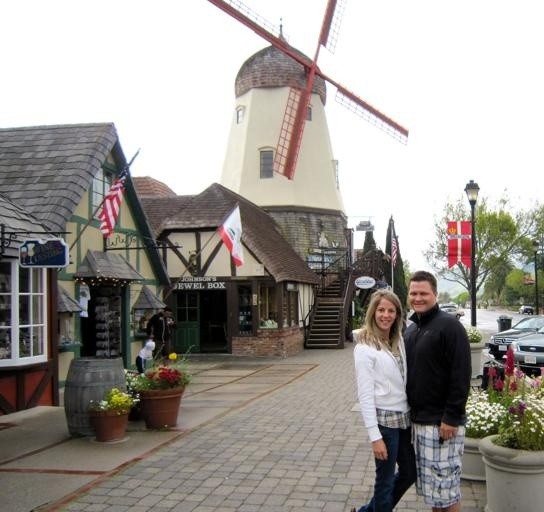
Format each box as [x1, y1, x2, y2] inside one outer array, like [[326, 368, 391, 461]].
[[464, 180, 480, 325], [532, 239, 540, 315]]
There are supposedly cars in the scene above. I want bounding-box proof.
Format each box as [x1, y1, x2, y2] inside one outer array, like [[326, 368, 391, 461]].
[[485, 315, 544, 359], [519, 305, 534, 315], [511, 326, 543, 373], [441, 308, 464, 319]]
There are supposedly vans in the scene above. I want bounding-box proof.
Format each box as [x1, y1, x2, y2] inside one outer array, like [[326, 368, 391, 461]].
[[440, 305, 456, 308]]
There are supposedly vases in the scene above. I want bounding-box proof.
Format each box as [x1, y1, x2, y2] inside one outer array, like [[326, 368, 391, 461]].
[[128, 402, 142, 420], [88, 408, 130, 443], [479, 435, 544, 512], [460, 438, 486, 481], [140, 388, 184, 427]]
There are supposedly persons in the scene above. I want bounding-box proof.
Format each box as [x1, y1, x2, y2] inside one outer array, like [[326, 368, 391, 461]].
[[401, 270, 472, 511], [351, 287, 416, 511], [147, 306, 177, 367], [136, 340, 154, 373]]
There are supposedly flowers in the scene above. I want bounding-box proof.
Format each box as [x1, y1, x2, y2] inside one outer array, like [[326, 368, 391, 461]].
[[488, 346, 544, 451], [126, 372, 139, 401], [464, 386, 506, 437], [88, 388, 133, 411], [132, 343, 192, 390]]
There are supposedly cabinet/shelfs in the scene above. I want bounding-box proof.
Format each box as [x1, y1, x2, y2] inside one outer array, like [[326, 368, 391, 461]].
[[237, 314, 253, 337]]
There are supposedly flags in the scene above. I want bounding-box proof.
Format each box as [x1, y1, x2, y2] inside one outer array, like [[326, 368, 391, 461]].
[[98, 167, 128, 239], [219, 206, 245, 267]]
